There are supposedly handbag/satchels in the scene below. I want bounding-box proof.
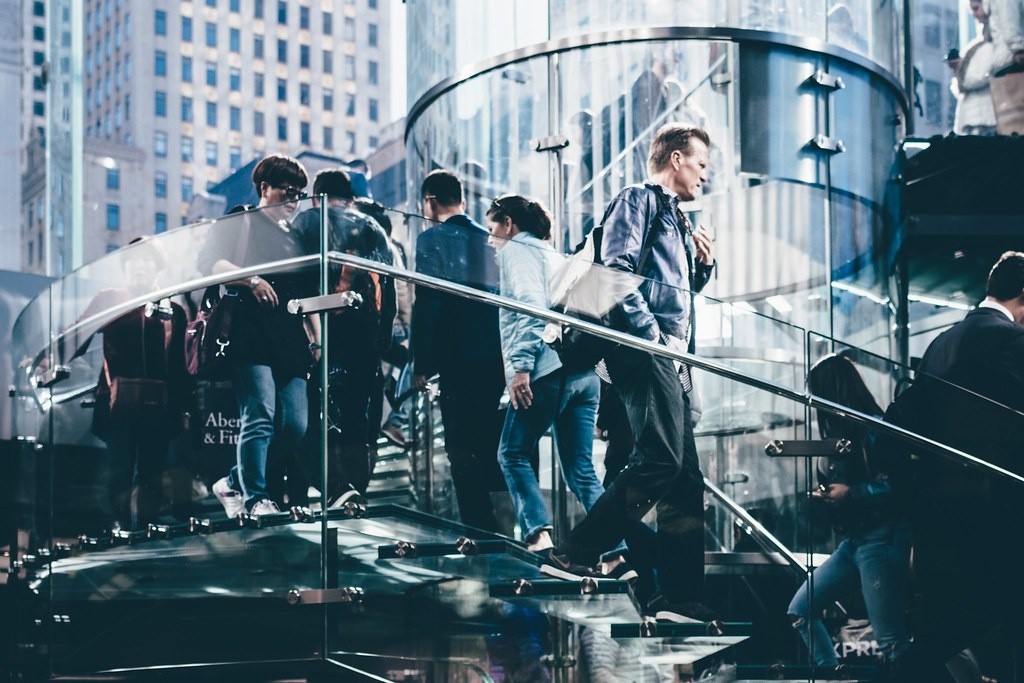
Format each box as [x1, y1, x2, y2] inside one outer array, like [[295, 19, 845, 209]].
[[875, 371, 958, 488], [183, 278, 247, 383], [559, 185, 657, 375]]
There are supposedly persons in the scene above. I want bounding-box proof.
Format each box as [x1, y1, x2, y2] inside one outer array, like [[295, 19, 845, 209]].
[[827, 4, 867, 53], [486, 195, 639, 586], [412, 170, 514, 540], [539, 124, 717, 622], [19, 153, 412, 530], [564, 41, 680, 256], [485, 599, 552, 683], [787, 356, 910, 664], [581, 599, 696, 683], [950, 0, 1024, 137], [909, 252, 1024, 683]]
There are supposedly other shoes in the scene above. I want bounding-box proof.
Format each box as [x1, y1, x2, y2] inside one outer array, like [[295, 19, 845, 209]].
[[525, 530, 556, 554], [600, 557, 639, 586], [242, 494, 291, 520], [211, 477, 250, 520]]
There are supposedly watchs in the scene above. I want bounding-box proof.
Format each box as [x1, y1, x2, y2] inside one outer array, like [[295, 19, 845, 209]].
[[249, 276, 262, 290]]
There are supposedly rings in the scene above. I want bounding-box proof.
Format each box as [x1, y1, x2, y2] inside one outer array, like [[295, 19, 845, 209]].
[[262, 296, 267, 300], [521, 390, 526, 394]]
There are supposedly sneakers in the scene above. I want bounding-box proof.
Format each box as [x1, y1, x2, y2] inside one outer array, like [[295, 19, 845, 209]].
[[540, 549, 617, 582], [655, 600, 728, 630]]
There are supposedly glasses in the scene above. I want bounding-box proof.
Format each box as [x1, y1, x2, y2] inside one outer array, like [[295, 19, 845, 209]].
[[268, 182, 307, 200]]
